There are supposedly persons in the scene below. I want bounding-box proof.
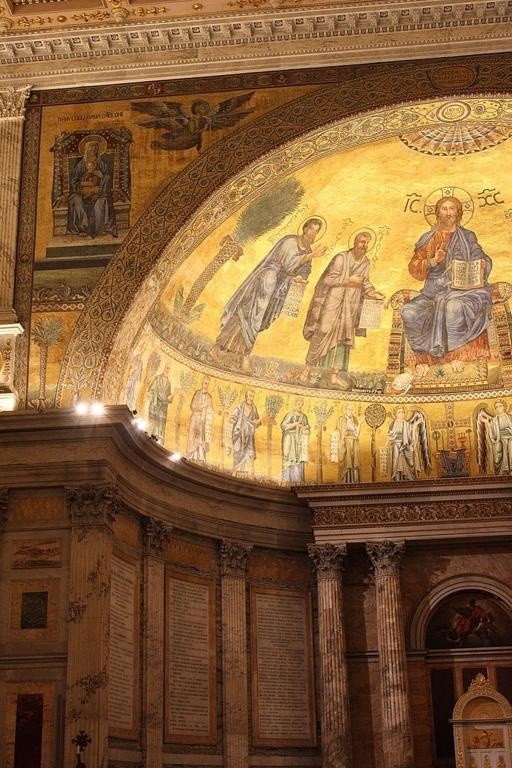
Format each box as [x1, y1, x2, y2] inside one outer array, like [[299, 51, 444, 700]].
[[67, 139, 115, 236], [186, 375, 215, 466], [383, 406, 425, 482], [336, 402, 371, 486], [397, 197, 494, 378], [444, 597, 495, 647], [228, 387, 263, 478], [277, 398, 312, 490], [145, 359, 171, 445], [481, 400, 512, 478], [300, 232, 387, 390], [213, 216, 328, 374], [149, 100, 211, 156], [123, 341, 148, 414]]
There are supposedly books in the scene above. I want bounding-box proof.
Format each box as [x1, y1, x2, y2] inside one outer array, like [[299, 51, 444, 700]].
[[449, 258, 486, 291]]
